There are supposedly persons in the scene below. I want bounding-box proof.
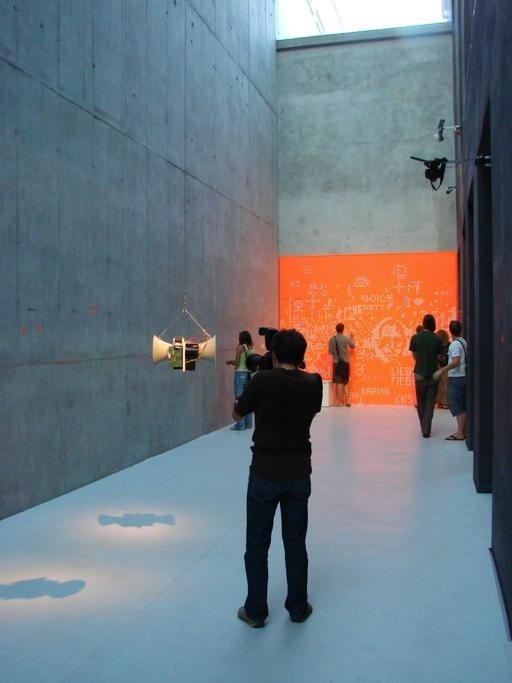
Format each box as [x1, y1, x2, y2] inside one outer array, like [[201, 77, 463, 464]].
[[232, 330, 323, 629], [409, 314, 468, 441], [258, 327, 277, 371], [225, 330, 256, 430], [328, 323, 355, 407]]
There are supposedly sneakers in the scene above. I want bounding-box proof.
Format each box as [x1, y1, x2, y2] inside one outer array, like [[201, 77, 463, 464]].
[[291, 602, 312, 621], [239, 607, 264, 627], [423, 434, 429, 438]]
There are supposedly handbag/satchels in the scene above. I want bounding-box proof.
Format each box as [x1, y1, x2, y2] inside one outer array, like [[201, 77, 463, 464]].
[[337, 359, 347, 378]]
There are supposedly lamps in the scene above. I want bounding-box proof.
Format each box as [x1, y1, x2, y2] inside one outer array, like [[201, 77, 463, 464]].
[[433, 119, 461, 142]]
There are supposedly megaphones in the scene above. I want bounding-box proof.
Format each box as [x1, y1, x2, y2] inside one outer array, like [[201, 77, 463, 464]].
[[152, 335, 183, 369], [185, 335, 217, 370]]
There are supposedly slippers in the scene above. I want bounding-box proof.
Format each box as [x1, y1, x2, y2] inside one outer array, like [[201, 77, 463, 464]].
[[446, 435, 463, 440]]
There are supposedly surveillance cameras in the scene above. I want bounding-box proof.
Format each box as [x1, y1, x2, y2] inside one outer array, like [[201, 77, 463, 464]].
[[447, 189, 453, 194]]
